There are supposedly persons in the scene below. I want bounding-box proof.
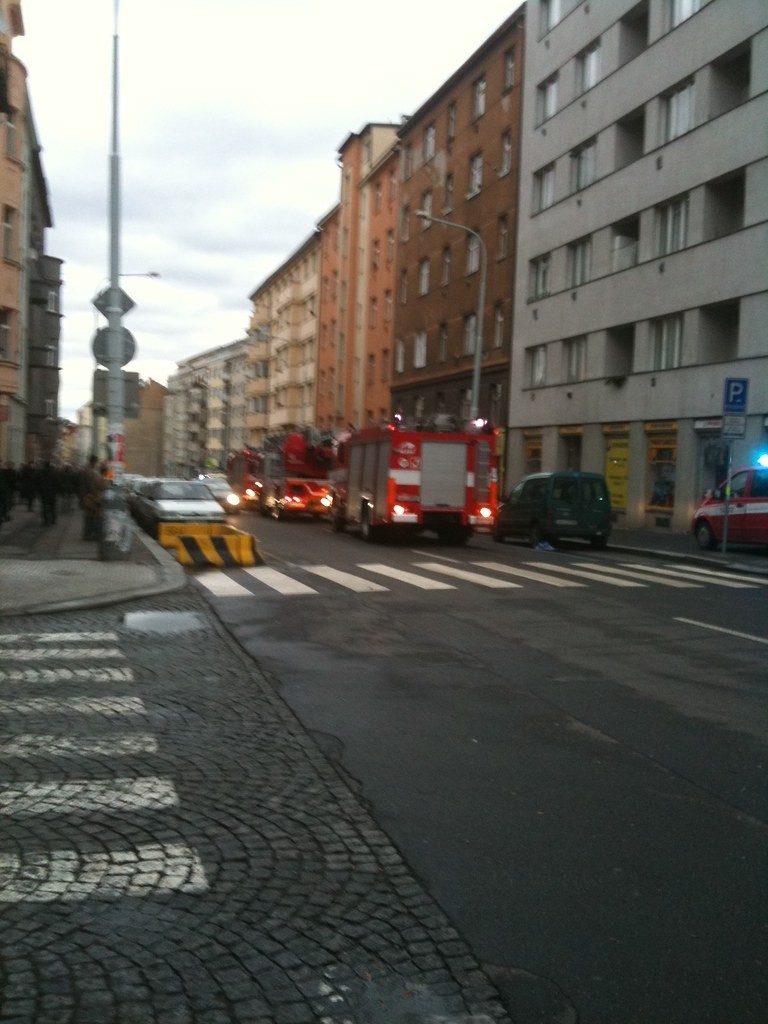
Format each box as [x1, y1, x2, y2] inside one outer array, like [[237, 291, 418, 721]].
[[0, 455, 115, 544]]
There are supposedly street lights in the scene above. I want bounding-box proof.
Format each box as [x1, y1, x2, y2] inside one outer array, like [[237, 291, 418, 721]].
[[417, 212, 487, 418]]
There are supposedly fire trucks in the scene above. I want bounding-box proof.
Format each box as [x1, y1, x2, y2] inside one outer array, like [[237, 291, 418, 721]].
[[226, 444, 262, 511], [325, 419, 501, 547], [258, 424, 335, 518]]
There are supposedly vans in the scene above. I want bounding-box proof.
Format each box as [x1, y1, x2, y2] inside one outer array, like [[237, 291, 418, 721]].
[[496, 471, 613, 552], [692, 466, 768, 551]]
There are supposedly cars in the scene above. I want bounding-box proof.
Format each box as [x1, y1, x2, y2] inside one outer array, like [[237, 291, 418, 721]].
[[128, 478, 226, 538], [195, 477, 239, 513]]
